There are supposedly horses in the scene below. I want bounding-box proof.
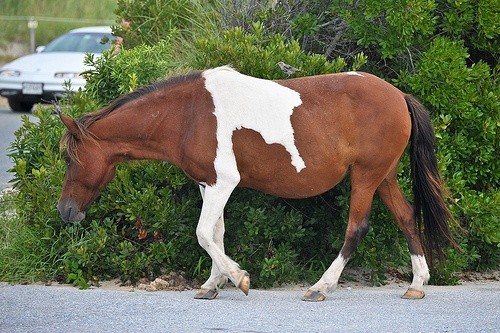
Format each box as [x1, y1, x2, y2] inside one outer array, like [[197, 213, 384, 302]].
[[53, 62, 468, 302]]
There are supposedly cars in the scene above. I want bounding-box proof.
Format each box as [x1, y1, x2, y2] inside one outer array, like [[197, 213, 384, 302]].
[[0, 26, 120, 113]]
